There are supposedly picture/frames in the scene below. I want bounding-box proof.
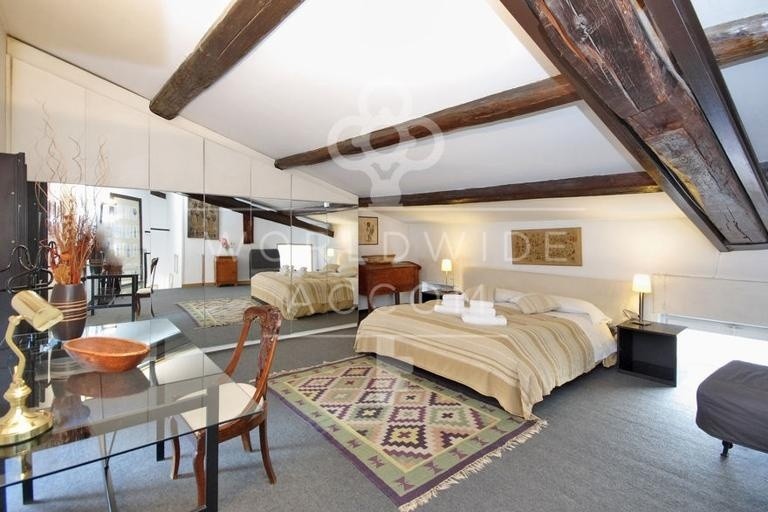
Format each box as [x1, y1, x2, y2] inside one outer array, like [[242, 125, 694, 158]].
[[358, 215, 379, 246]]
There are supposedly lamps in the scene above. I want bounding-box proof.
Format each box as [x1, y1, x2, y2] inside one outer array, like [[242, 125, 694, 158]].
[[440, 259, 453, 287], [0, 289, 66, 449], [326, 247, 335, 264], [630, 273, 653, 327]]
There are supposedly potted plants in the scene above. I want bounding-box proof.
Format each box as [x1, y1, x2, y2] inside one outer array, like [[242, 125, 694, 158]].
[[34, 194, 102, 342]]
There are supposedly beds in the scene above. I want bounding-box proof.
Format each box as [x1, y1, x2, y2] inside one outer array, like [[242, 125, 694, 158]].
[[250, 252, 358, 321], [354, 266, 639, 419]]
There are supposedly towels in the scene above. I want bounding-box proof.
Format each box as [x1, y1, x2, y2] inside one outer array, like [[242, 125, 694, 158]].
[[461, 315, 507, 327], [443, 294, 464, 307], [434, 305, 469, 314], [470, 300, 495, 316]]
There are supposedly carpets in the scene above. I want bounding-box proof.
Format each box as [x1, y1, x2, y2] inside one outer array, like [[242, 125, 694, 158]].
[[172, 294, 265, 330], [249, 352, 550, 512]]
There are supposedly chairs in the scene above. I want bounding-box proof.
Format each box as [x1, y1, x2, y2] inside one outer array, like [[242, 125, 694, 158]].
[[172, 305, 281, 512], [136, 256, 161, 318]]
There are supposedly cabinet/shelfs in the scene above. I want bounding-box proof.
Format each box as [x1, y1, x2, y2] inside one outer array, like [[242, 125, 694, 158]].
[[213, 255, 239, 288], [359, 260, 421, 309]]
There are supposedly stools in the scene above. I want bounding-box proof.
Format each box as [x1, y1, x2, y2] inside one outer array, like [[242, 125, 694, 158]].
[[696, 360, 768, 457]]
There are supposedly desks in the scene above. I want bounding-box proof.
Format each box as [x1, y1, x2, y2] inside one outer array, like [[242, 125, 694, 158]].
[[88, 272, 139, 333]]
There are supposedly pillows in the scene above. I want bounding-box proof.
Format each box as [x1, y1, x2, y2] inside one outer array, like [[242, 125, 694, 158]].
[[464, 286, 520, 303], [508, 292, 610, 326], [514, 294, 562, 315]]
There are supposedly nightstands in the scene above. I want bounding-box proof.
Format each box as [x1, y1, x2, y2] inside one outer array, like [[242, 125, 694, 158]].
[[615, 317, 688, 388], [413, 287, 461, 304]]
